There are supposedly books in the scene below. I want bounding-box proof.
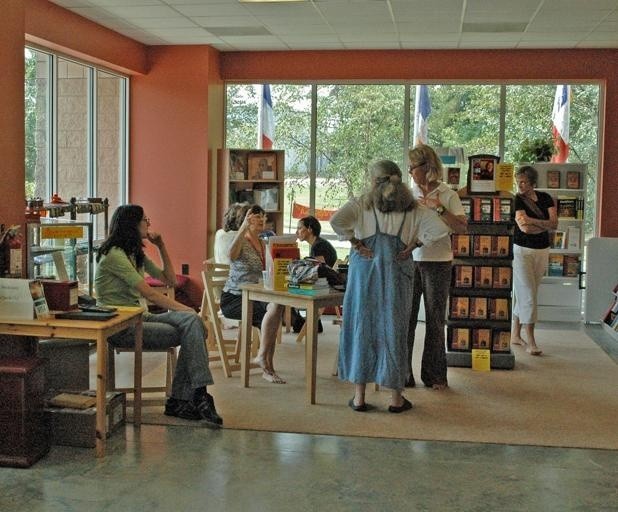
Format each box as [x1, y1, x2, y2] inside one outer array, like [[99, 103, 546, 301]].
[[543, 196, 584, 277], [284, 277, 330, 296], [603, 284, 618, 332], [51, 393, 95, 409], [451, 199, 512, 352]]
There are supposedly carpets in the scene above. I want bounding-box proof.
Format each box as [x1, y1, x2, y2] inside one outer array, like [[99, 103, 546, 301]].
[[117, 315, 617, 453]]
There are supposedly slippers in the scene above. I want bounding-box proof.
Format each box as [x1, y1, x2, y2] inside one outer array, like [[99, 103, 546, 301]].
[[349, 396, 368, 412], [389, 396, 412, 413]]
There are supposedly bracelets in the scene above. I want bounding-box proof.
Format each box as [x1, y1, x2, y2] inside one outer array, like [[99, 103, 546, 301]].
[[436, 206, 446, 215], [415, 243, 422, 247], [352, 240, 361, 249]]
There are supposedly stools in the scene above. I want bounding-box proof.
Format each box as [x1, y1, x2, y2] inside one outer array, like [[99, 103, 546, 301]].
[[0, 359, 52, 469], [39, 339, 90, 391]]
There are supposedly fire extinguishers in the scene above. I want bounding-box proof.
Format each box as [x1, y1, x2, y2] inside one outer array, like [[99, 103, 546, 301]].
[[0, 224, 23, 279]]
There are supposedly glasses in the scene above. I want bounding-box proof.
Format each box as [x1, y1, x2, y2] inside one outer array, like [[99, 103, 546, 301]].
[[407, 162, 427, 173]]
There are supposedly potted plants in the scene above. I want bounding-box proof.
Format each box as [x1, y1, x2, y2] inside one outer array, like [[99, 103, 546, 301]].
[[512, 131, 562, 163]]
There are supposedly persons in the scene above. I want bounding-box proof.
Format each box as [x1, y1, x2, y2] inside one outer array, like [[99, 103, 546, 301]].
[[220, 205, 286, 384], [512, 166, 557, 355], [282, 216, 336, 332], [252, 159, 272, 179], [212, 203, 251, 302], [479, 161, 494, 180], [95, 205, 223, 424], [405, 145, 468, 390], [330, 160, 449, 412]]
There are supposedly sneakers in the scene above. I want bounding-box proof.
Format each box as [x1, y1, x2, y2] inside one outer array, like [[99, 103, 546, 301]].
[[164, 399, 203, 421], [189, 391, 223, 425]]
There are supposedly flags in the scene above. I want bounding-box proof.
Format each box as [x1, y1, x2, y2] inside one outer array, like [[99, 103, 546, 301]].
[[413, 85, 430, 150], [258, 84, 274, 149], [551, 84, 571, 163]]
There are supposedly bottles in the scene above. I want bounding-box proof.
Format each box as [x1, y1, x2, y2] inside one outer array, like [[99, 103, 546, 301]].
[[25, 196, 44, 208]]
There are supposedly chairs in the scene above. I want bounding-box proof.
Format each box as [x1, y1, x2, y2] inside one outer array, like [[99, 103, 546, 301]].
[[107, 285, 180, 409], [295, 304, 342, 340], [199, 268, 264, 381], [202, 260, 264, 360]]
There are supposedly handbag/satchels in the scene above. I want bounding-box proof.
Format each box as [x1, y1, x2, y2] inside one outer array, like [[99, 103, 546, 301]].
[[318, 262, 348, 293]]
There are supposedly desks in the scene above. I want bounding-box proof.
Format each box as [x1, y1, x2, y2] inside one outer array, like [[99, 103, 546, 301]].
[[0, 305, 147, 460], [239, 284, 346, 403]]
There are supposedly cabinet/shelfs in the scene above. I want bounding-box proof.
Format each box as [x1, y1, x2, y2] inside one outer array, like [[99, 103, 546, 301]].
[[514, 162, 588, 324], [445, 187, 516, 371], [216, 148, 285, 238], [26, 196, 110, 299]]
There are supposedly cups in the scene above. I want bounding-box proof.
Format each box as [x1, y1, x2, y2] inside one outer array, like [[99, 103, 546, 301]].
[[262, 271, 272, 289], [182, 264, 189, 275]]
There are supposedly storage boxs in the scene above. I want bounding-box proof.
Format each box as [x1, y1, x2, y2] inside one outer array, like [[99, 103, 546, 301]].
[[35, 280, 79, 309], [76, 385, 127, 440], [43, 399, 96, 449]]
